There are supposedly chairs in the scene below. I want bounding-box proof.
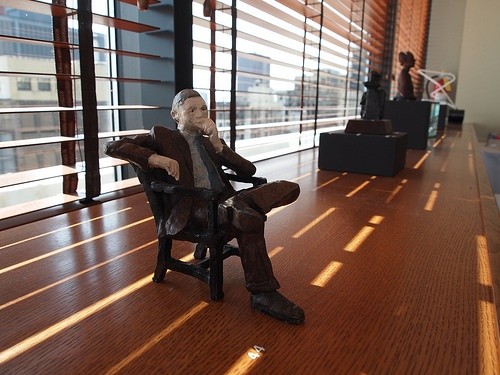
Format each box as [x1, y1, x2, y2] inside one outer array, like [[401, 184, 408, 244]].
[[128, 159, 267, 301]]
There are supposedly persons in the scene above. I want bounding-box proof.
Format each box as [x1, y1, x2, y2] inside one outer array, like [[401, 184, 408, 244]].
[[396, 51, 417, 98], [360, 70, 386, 120], [104, 89, 305, 323]]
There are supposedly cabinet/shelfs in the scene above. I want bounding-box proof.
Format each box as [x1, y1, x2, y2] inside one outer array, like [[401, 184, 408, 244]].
[[383, 98, 440, 151], [318, 128, 405, 177]]
